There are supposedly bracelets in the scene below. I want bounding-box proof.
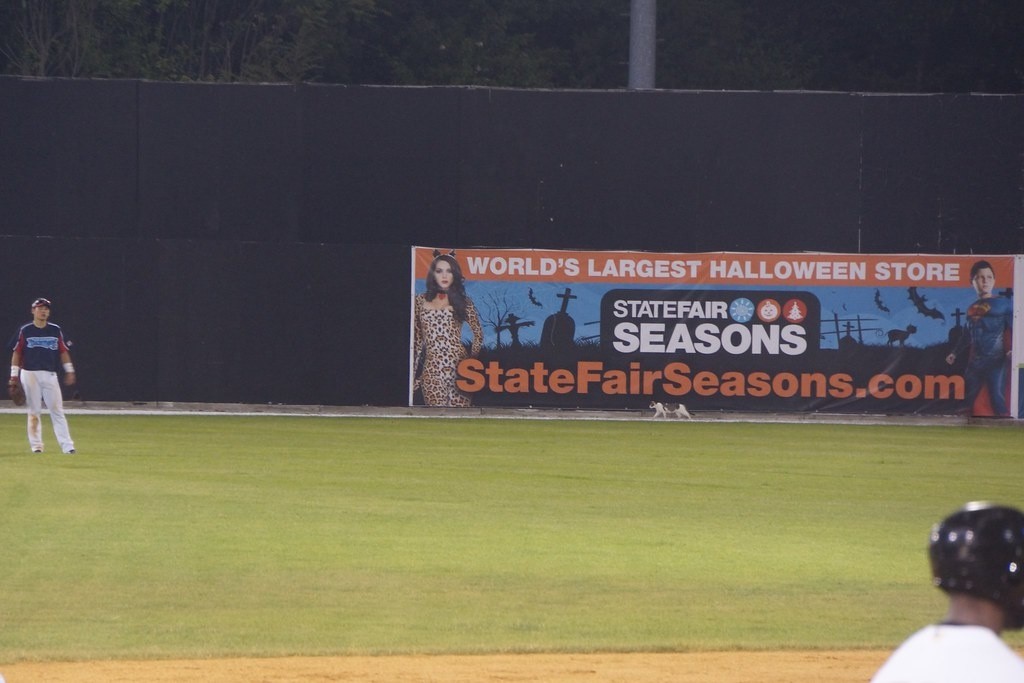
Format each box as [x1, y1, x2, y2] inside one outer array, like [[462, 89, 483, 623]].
[[10, 365, 21, 378], [62, 362, 75, 374]]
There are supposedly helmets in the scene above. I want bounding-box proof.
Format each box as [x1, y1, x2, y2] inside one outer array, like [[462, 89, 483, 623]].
[[928, 502, 1024, 630]]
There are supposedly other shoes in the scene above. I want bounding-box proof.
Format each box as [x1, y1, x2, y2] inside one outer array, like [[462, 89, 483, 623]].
[[34, 450, 40, 453], [67, 450, 76, 454]]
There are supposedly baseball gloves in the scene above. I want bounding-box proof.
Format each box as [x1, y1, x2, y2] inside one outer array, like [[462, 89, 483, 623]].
[[6, 379, 26, 406]]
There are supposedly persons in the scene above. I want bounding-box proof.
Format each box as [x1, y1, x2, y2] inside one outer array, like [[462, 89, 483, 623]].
[[9, 298, 76, 455], [945, 261, 1014, 417], [870, 504, 1024, 683], [414, 254, 483, 408]]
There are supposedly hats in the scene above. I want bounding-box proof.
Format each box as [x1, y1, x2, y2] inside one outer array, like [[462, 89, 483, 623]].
[[32, 298, 51, 307]]
